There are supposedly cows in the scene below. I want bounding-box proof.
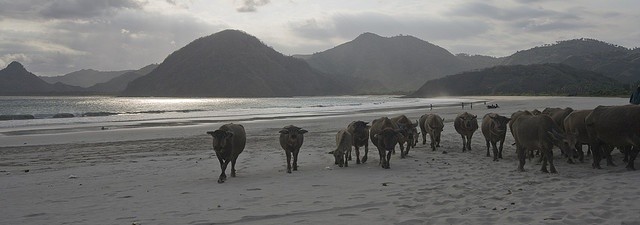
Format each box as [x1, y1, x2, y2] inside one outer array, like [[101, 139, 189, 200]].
[[370, 117, 405, 169], [420, 114, 444, 150], [347, 121, 371, 163], [542, 107, 573, 126], [391, 115, 418, 158], [509, 110, 580, 173], [278, 125, 308, 173], [455, 112, 478, 151], [481, 113, 511, 161], [585, 105, 640, 171], [564, 110, 596, 163], [328, 130, 352, 168], [206, 123, 246, 183]]
